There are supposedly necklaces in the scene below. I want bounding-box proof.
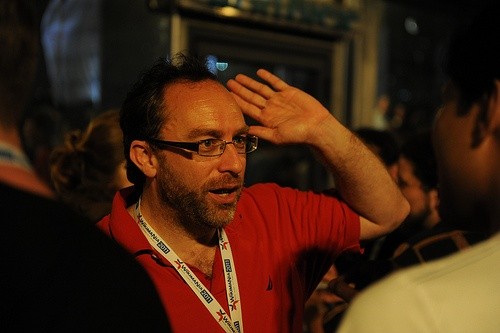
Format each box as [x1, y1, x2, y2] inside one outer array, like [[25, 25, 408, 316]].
[[134, 193, 244, 333]]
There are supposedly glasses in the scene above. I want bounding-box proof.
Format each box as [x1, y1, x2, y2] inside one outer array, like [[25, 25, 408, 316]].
[[145, 134, 258, 157]]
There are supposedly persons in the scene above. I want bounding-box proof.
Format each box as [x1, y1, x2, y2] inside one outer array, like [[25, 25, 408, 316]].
[[335, 0, 499, 333], [0, 1, 176, 333], [23, 109, 485, 333], [94, 51, 412, 333]]
